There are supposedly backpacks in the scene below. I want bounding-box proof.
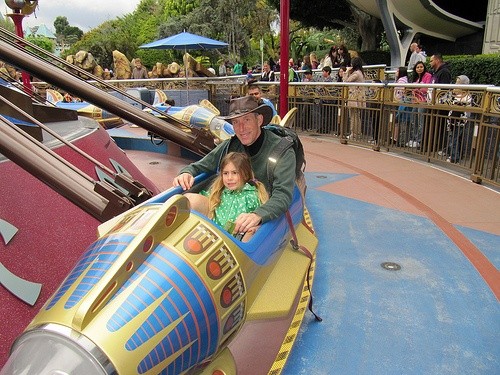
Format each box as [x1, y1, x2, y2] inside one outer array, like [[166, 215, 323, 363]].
[[226, 124, 306, 186]]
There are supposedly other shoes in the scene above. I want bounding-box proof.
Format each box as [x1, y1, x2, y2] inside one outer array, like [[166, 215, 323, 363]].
[[406, 140, 420, 148], [438, 151, 450, 156], [447, 157, 453, 163], [346, 134, 361, 139], [368, 139, 376, 143], [385, 137, 396, 144]]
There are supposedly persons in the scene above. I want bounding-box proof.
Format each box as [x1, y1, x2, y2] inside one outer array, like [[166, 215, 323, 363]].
[[288, 45, 366, 140], [207, 152, 269, 241], [248, 86, 278, 125], [368, 42, 475, 164], [131, 58, 149, 88], [62, 93, 84, 103], [207, 56, 280, 103], [172, 96, 297, 243]]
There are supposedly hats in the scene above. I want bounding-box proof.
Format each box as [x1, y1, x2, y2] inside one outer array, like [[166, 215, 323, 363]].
[[303, 69, 312, 74], [217, 96, 273, 128]]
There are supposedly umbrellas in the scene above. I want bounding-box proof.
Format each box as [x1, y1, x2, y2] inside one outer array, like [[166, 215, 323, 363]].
[[138, 29, 229, 106]]
